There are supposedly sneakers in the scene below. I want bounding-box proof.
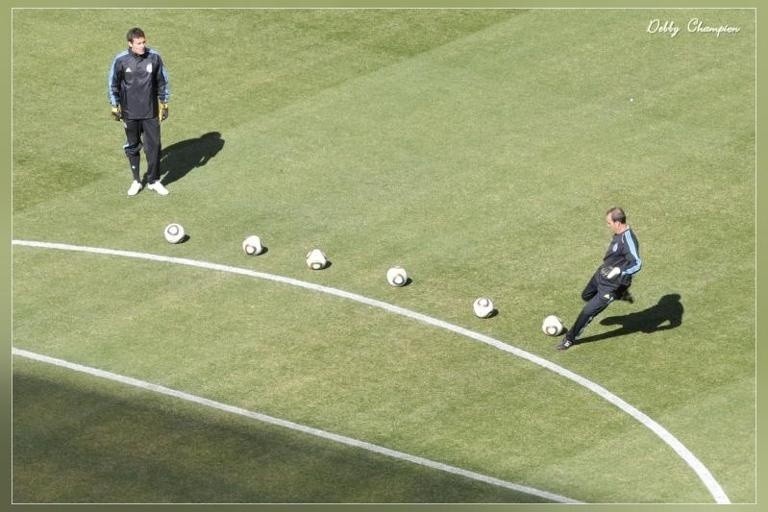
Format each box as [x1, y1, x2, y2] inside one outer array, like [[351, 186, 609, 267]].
[[557, 336, 575, 350], [146, 180, 170, 196], [127, 180, 143, 196], [619, 290, 633, 303]]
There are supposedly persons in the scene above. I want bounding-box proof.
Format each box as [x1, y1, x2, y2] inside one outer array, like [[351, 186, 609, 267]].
[[557, 208, 642, 350], [108, 28, 169, 196]]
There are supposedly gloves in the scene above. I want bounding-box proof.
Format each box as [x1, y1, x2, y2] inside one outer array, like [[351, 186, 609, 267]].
[[600, 266, 622, 280], [112, 104, 123, 122], [158, 101, 168, 122]]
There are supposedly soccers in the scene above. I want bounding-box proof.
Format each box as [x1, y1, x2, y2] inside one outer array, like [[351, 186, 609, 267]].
[[242, 235, 262, 256], [474, 298, 494, 317], [541, 315, 565, 336], [386, 264, 409, 288], [305, 248, 329, 271], [164, 223, 186, 244]]
